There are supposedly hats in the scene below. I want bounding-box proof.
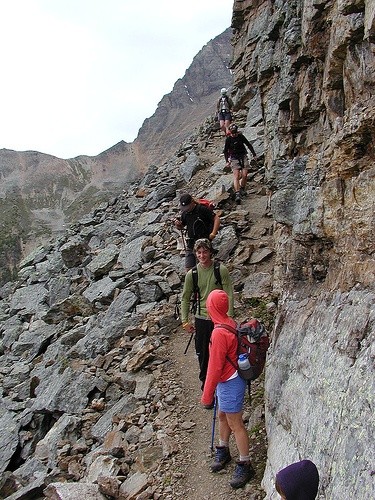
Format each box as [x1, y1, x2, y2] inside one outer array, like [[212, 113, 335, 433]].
[[221, 88, 228, 93], [276, 460, 320, 500], [194, 238, 211, 251], [178, 194, 192, 210]]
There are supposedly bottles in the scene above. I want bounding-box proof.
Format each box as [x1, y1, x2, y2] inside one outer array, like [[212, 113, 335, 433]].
[[237, 354, 250, 370]]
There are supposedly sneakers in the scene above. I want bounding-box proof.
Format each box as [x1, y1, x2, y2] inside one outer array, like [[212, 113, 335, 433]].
[[211, 446, 232, 471], [235, 192, 241, 205], [230, 460, 256, 488], [240, 188, 247, 196]]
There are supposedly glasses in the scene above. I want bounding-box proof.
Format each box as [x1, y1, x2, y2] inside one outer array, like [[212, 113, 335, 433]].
[[229, 125, 238, 130]]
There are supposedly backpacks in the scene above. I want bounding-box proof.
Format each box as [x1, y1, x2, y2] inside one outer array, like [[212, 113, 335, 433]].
[[220, 318, 270, 380], [220, 96, 230, 114], [197, 199, 215, 211]]
